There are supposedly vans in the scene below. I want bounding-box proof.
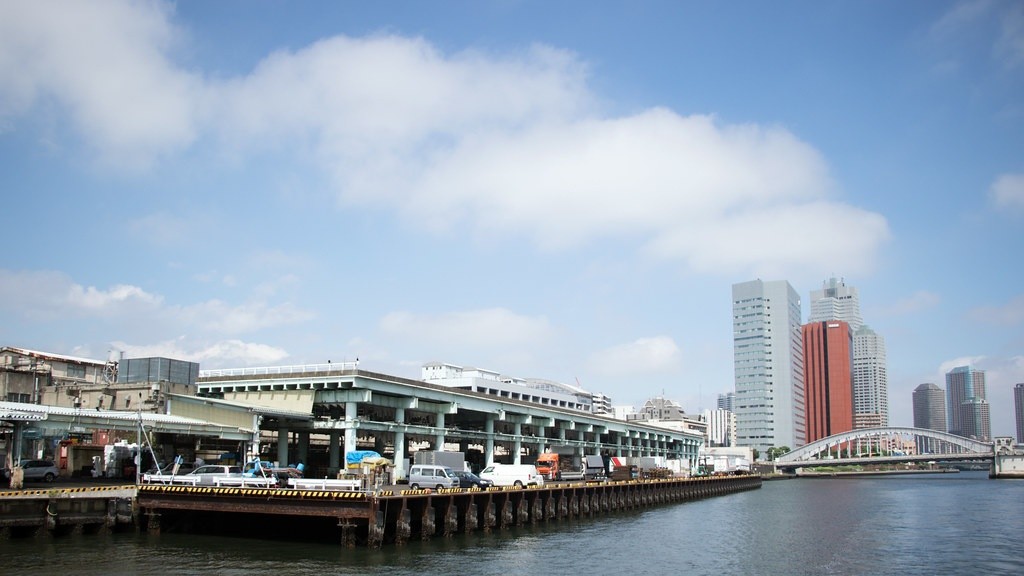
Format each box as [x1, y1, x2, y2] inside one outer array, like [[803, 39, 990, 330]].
[[408, 464, 461, 492], [182, 464, 242, 486]]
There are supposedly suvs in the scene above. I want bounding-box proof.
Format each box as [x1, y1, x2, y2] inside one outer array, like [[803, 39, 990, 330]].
[[0, 459, 59, 483]]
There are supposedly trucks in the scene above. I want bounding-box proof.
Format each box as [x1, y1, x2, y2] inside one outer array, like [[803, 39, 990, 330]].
[[479, 463, 544, 489], [536, 453, 562, 481], [242, 461, 304, 488]]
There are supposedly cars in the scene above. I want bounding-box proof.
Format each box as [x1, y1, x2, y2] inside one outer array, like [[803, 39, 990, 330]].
[[440, 470, 493, 490]]
